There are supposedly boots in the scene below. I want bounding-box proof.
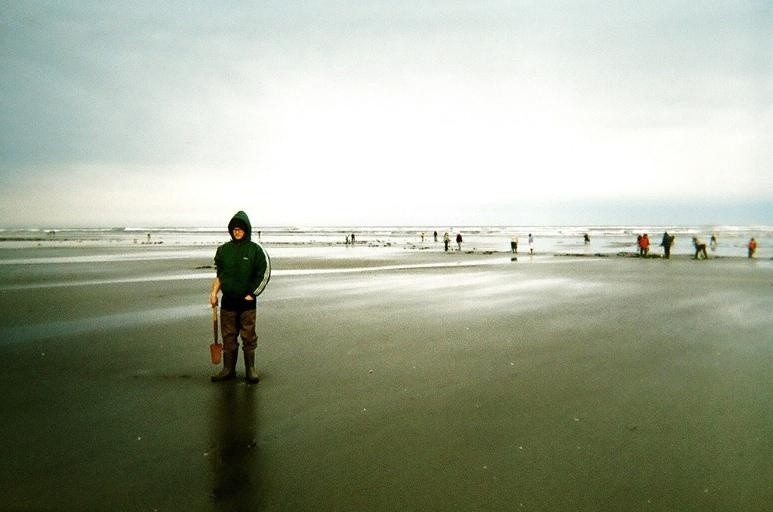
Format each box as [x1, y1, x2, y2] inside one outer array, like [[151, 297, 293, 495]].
[[210, 348, 239, 382], [244, 351, 260, 383]]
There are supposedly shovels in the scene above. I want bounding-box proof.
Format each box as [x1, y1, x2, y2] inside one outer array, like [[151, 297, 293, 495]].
[[210, 294, 222, 365]]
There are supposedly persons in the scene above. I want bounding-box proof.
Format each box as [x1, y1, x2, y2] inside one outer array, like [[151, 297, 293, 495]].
[[350, 232, 354, 243], [209, 212, 271, 383], [639, 233, 650, 255], [420, 232, 424, 243], [583, 233, 590, 243], [454, 231, 463, 251], [636, 233, 643, 255], [510, 233, 519, 253], [443, 231, 451, 252], [657, 231, 674, 260], [527, 233, 535, 253], [709, 231, 718, 245], [747, 237, 757, 258], [433, 230, 437, 241], [691, 236, 708, 260]]
